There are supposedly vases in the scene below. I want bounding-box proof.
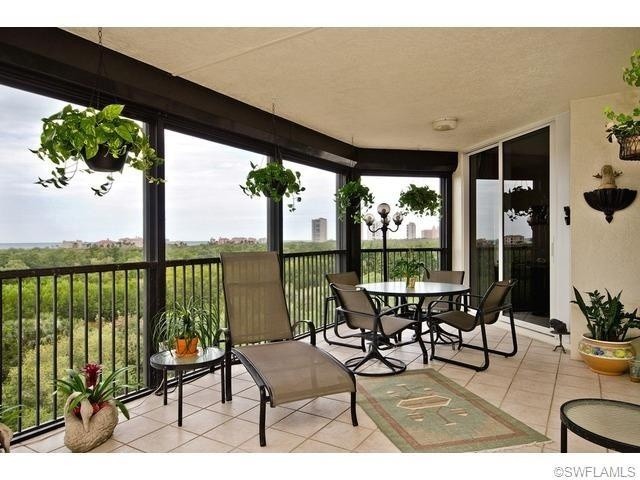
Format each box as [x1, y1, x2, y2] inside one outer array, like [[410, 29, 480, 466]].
[[62, 391, 119, 452]]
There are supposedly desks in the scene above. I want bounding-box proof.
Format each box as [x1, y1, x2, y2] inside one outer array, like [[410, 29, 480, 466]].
[[357, 276, 470, 364], [559, 398, 640, 452], [149, 343, 225, 428]]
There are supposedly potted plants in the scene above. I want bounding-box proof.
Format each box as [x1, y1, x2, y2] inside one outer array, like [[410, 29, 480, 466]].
[[390, 259, 431, 288], [569, 284, 640, 377], [239, 159, 305, 214], [149, 294, 220, 357], [334, 180, 375, 226], [601, 46, 640, 161], [29, 101, 167, 197], [394, 183, 445, 220], [503, 184, 545, 222]]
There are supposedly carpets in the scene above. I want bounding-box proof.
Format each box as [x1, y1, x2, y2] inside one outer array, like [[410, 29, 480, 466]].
[[351, 368, 553, 452]]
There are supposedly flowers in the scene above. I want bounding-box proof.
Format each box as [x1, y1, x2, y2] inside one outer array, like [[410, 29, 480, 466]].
[[48, 362, 141, 422]]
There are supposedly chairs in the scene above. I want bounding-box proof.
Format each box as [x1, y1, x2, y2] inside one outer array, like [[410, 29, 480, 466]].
[[321, 269, 387, 347], [331, 282, 424, 377], [427, 278, 521, 372], [409, 270, 465, 344], [218, 249, 359, 448]]
